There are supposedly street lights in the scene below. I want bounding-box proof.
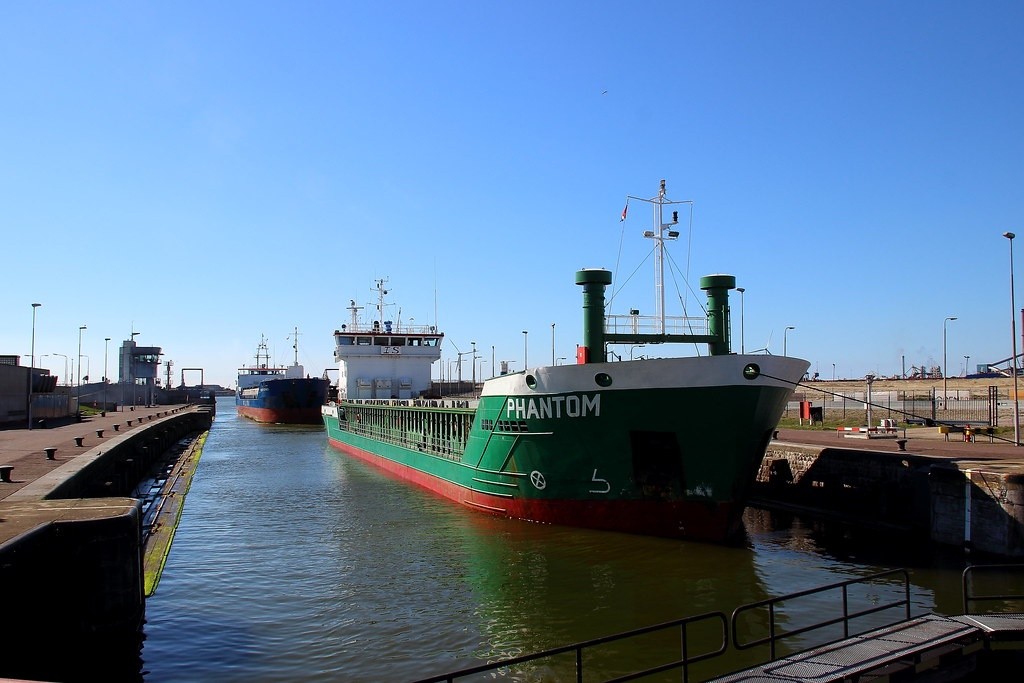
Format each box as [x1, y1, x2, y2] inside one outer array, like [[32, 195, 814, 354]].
[[783, 326, 795, 357], [943, 317, 957, 410], [131, 332, 140, 411], [1002, 230, 1020, 448], [104, 338, 110, 413], [76, 326, 88, 415], [27, 303, 42, 430]]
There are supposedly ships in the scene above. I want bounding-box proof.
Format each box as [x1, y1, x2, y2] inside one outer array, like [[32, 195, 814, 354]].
[[321, 176, 813, 551], [233, 331, 332, 425]]
